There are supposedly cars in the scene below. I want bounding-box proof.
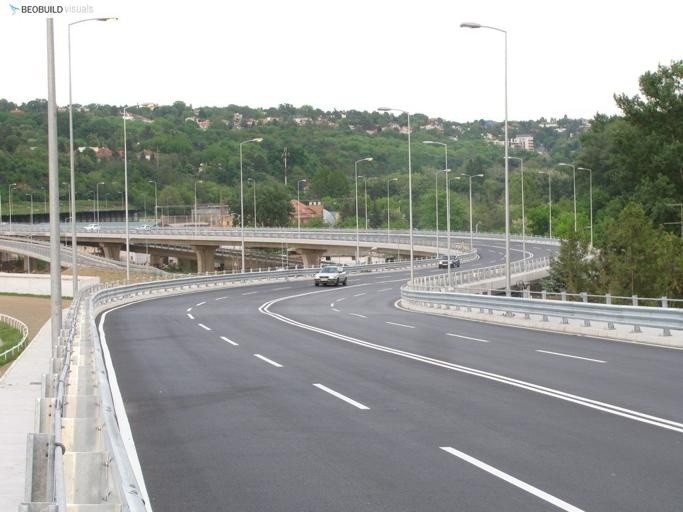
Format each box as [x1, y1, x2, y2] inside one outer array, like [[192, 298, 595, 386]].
[[315, 265, 348, 287]]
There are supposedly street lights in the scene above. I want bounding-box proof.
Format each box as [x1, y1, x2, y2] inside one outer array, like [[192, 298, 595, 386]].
[[65, 16, 119, 302], [352, 107, 485, 288], [558, 163, 577, 233], [502, 156, 526, 273], [6, 178, 206, 238], [578, 168, 593, 250], [459, 22, 511, 297], [538, 170, 552, 239], [238, 137, 263, 272], [295, 179, 308, 235], [246, 177, 258, 230]]
